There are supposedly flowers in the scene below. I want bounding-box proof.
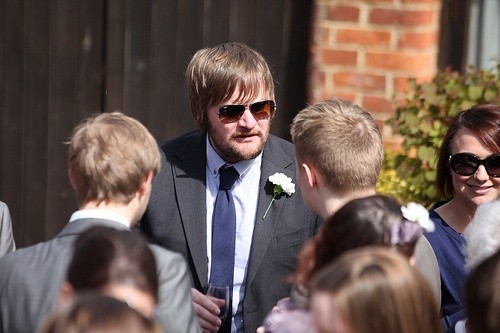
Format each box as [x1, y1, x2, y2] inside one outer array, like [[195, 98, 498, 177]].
[[263, 172, 296, 218], [401, 201, 435, 233]]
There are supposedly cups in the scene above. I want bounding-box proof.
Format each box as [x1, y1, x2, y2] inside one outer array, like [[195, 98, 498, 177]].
[[206, 284, 230, 327]]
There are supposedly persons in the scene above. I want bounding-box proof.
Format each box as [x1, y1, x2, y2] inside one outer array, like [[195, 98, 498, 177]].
[[422, 103, 500, 333], [0, 111, 202, 333], [36, 226, 166, 333], [463, 200, 500, 333], [0, 201, 16, 259], [256, 98, 443, 333], [130, 41, 324, 333]]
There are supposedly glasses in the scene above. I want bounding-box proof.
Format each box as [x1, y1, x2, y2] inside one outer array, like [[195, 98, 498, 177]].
[[448, 152, 500, 177], [207, 99, 276, 123]]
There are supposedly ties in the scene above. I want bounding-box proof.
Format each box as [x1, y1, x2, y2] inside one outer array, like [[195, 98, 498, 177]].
[[209, 164, 240, 333]]
